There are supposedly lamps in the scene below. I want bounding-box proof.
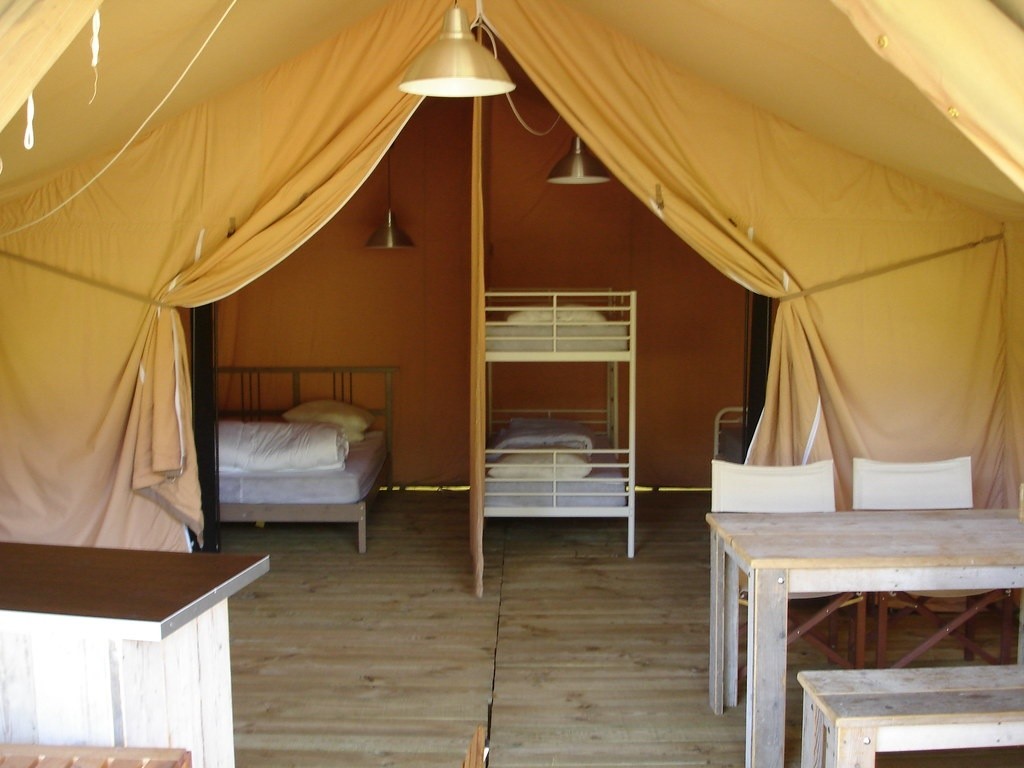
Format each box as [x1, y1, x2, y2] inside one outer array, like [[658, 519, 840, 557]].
[[398, 0, 516, 98], [367, 146, 415, 250], [547, 131, 612, 186]]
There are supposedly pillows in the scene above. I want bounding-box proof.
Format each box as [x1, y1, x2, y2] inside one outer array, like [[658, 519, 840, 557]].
[[342, 430, 366, 442], [507, 310, 607, 325], [486, 447, 593, 480], [280, 398, 377, 432]]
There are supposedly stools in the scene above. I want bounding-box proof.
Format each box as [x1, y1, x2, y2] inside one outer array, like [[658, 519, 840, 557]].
[[0, 743, 197, 768]]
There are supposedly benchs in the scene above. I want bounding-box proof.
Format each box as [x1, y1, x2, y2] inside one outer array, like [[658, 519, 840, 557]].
[[797, 664, 1024, 768]]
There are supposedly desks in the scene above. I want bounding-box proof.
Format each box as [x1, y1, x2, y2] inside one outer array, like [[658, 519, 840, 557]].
[[0, 542, 273, 768], [705, 511, 1024, 768]]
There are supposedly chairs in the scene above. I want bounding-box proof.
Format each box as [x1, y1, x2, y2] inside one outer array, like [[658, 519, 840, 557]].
[[852, 455, 1018, 668], [711, 456, 867, 691]]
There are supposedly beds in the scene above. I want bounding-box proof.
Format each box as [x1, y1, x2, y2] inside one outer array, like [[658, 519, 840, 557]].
[[216, 364, 399, 555], [483, 292, 637, 559]]
